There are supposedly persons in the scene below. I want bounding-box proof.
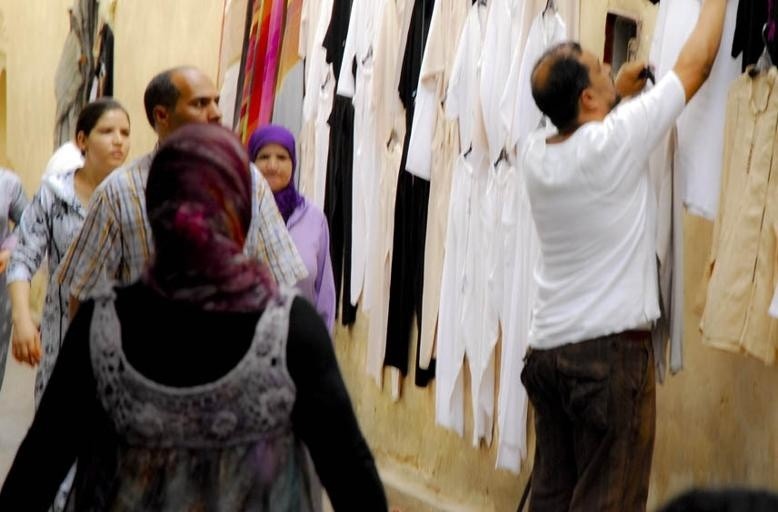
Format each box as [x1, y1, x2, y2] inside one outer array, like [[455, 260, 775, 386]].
[[0, 165, 31, 396], [58, 65, 310, 322], [518, 0, 730, 512], [0, 97, 130, 413], [659, 486, 778, 512], [246, 124, 337, 336], [0, 123, 391, 511]]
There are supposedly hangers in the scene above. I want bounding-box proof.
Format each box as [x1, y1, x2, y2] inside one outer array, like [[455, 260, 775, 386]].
[[725, 21, 778, 114], [458, 0, 568, 43]]
